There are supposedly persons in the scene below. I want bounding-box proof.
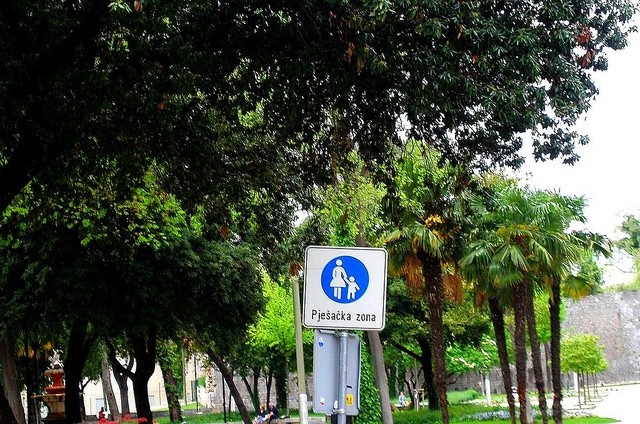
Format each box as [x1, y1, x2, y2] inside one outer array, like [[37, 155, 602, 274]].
[[267, 402, 279, 424], [399, 392, 407, 407], [99, 407, 104, 420], [255, 404, 267, 424], [40, 401, 51, 424]]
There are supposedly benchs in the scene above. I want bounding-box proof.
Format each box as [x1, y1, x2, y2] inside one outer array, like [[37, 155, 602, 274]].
[[261, 415, 324, 423]]
